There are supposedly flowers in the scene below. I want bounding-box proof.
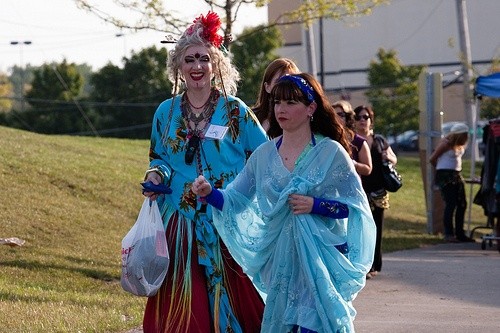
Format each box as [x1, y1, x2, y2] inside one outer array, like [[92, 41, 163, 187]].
[[188, 10, 232, 49]]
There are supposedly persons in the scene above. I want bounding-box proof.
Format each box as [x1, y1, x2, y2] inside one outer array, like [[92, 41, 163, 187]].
[[352, 106, 397, 280], [247, 57, 301, 140], [143, 11, 272, 332], [330, 101, 373, 176], [191, 73, 376, 333], [429, 124, 476, 244]]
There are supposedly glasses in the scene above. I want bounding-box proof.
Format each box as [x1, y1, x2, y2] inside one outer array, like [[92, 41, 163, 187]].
[[337, 112, 346, 117], [185, 135, 201, 165], [354, 115, 370, 121]]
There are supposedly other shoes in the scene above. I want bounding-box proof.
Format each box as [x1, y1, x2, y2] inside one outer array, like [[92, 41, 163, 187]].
[[366, 272, 378, 278], [445, 234, 460, 243], [455, 234, 475, 243]]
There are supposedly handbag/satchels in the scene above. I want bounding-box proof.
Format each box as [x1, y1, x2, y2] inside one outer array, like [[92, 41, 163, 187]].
[[382, 160, 402, 192]]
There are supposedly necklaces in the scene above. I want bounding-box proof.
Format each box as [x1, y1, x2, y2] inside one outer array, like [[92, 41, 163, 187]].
[[184, 89, 213, 109]]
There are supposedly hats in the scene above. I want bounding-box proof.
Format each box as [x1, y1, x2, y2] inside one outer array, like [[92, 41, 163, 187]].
[[450, 124, 469, 135]]
